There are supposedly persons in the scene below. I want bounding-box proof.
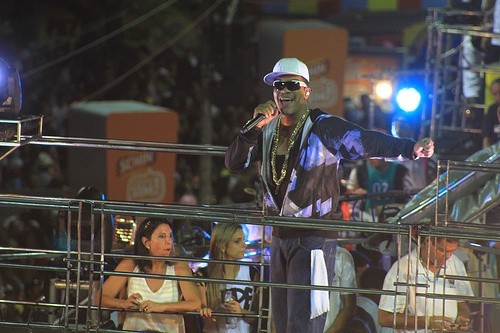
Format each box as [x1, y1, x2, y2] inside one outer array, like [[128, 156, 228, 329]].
[[0, 0, 271, 332], [225, 57, 434, 332], [324, 78, 500, 332]]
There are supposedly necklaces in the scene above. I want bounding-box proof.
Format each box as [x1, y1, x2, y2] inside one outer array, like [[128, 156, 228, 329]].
[[271, 105, 311, 185]]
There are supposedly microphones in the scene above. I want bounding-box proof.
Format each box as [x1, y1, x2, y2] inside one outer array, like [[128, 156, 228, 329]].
[[239, 105, 277, 135]]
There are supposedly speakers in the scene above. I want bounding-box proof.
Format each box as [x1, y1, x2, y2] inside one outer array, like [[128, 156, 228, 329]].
[[69, 100, 179, 241], [236, 19, 345, 122]]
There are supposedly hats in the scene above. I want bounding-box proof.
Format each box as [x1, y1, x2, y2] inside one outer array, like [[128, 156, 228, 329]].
[[263, 58, 310, 85], [392, 112, 415, 125]]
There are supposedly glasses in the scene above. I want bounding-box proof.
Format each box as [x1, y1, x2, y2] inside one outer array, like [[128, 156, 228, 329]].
[[273, 80, 307, 91]]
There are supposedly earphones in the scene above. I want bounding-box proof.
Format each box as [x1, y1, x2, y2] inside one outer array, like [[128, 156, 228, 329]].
[[305, 91, 310, 96]]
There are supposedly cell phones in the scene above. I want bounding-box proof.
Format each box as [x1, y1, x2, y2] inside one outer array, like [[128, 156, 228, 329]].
[[449, 322, 469, 327]]
